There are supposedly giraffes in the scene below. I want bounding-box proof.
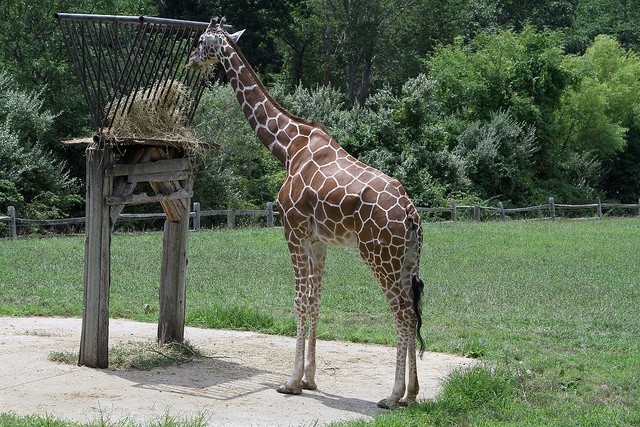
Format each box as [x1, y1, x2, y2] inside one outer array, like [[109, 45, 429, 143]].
[[190, 16, 428, 411]]
